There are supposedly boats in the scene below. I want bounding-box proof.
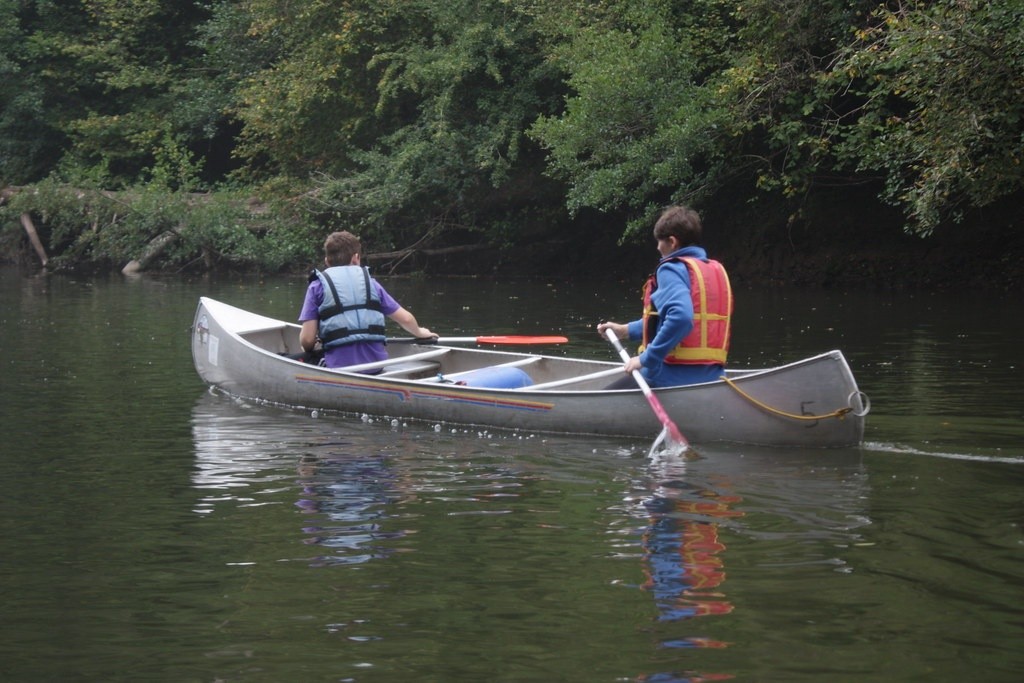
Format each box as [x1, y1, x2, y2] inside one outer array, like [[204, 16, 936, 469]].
[[190, 296, 867, 451]]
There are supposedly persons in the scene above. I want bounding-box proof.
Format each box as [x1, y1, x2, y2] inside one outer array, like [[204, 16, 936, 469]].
[[597, 206, 733, 390], [298, 231, 439, 375]]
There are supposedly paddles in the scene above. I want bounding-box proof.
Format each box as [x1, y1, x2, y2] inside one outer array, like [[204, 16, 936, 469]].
[[387, 333, 571, 347], [606, 325, 690, 452]]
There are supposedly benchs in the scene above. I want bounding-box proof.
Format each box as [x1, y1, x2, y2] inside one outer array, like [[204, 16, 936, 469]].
[[374, 359, 441, 376]]
